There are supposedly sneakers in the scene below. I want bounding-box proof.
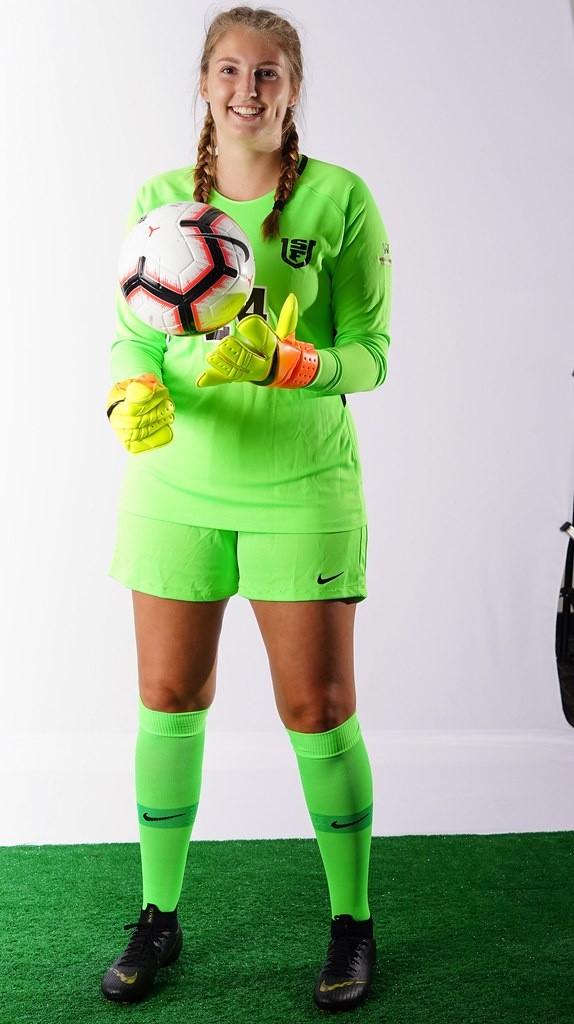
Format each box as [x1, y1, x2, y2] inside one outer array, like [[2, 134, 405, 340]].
[[313, 913, 377, 1012], [101, 903, 183, 1000]]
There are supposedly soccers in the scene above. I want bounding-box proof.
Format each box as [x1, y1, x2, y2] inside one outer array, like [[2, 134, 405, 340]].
[[122, 202, 255, 337]]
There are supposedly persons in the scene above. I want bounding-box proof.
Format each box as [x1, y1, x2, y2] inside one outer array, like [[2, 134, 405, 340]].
[[100, 6, 393, 1010]]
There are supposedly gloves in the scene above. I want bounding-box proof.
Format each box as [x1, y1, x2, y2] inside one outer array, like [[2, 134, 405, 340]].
[[106, 372, 177, 454], [194, 293, 299, 386]]
